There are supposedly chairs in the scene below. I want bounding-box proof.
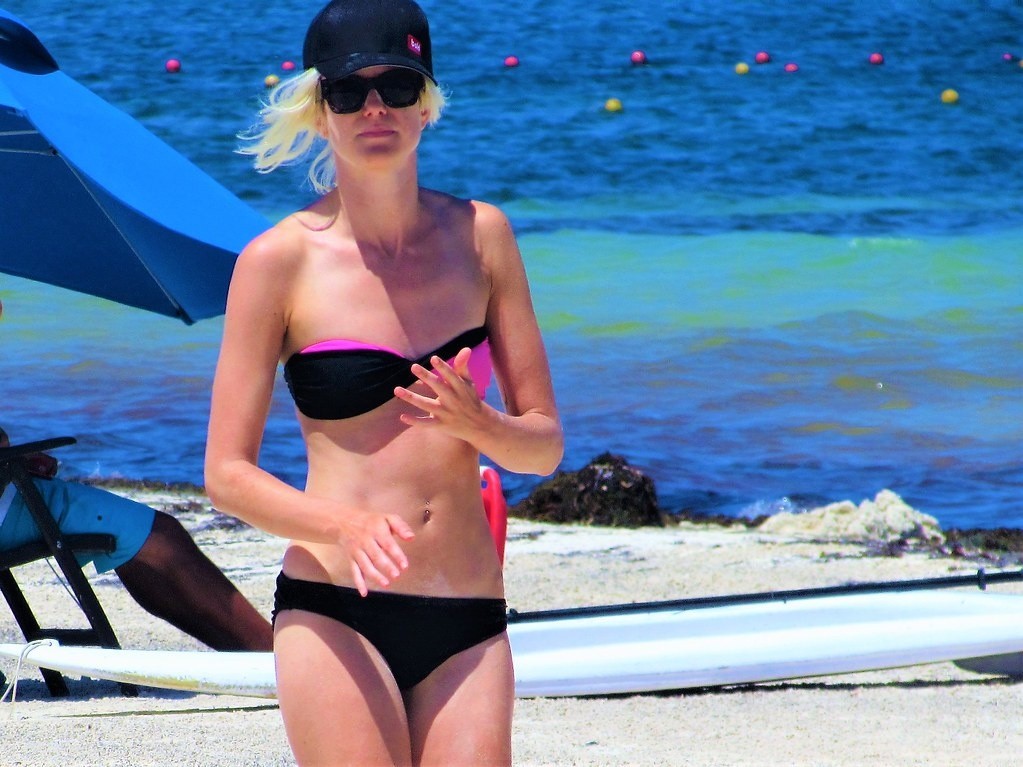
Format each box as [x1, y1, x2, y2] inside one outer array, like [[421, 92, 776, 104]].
[[0, 428, 139, 700]]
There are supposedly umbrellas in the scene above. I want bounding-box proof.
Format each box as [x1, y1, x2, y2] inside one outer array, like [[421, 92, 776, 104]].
[[0, 7, 274, 327]]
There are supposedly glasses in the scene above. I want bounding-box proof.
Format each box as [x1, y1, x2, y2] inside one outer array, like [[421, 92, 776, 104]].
[[321, 68, 425, 114]]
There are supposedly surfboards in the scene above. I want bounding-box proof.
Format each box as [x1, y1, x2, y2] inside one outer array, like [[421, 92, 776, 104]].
[[2, 567, 1023, 698]]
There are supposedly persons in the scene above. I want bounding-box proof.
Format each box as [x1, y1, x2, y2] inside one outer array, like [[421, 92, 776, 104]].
[[0, 428, 274, 653], [204, 0, 565, 767]]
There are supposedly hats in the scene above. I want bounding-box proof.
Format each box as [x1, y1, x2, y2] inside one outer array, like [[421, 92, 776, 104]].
[[303, 0, 438, 87]]
